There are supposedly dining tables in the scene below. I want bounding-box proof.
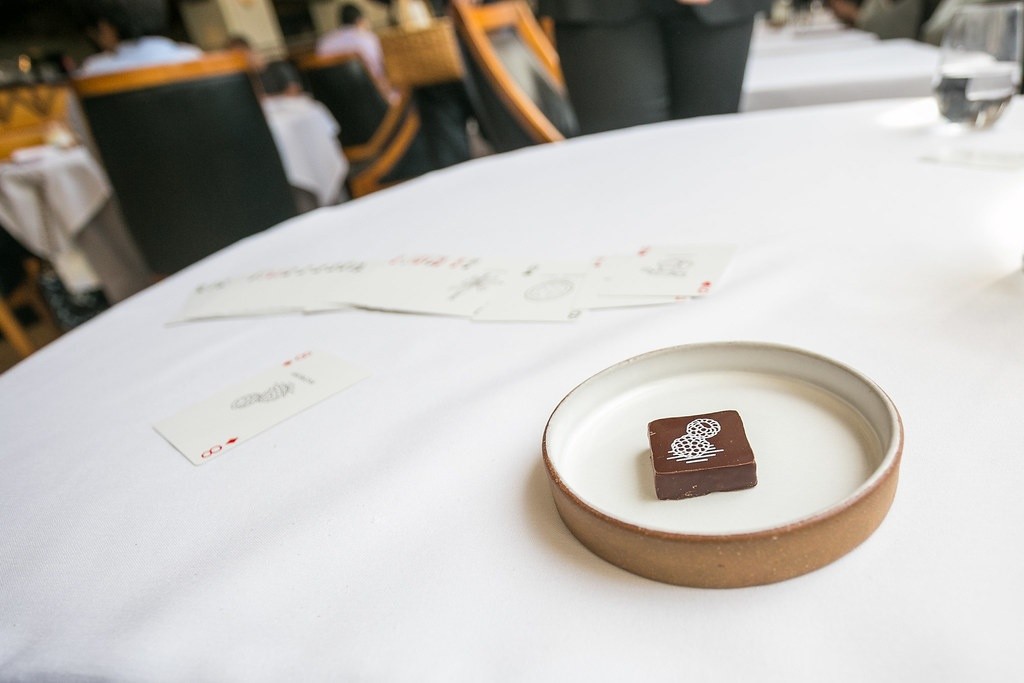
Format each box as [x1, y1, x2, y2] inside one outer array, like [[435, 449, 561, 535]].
[[0, 97, 1024, 680], [0, 95, 349, 305]]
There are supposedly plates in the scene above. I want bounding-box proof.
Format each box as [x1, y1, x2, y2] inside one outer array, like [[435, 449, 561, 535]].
[[543, 340, 908, 591]]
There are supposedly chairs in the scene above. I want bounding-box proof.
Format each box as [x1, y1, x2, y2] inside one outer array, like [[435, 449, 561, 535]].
[[77, 48, 302, 285], [453, 0, 581, 153], [342, 16, 472, 199], [296, 53, 392, 146]]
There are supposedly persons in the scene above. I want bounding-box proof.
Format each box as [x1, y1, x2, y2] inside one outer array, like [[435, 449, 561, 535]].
[[260, 62, 302, 99], [68, 0, 203, 138], [315, 5, 387, 76], [829, 0, 921, 39], [538, 0, 757, 136]]
